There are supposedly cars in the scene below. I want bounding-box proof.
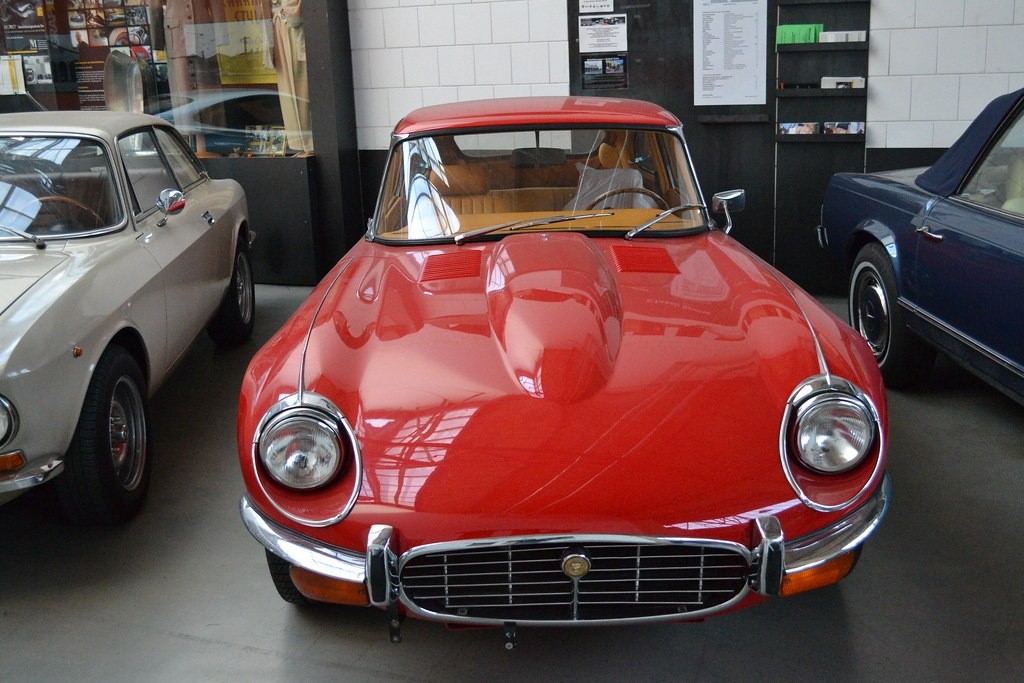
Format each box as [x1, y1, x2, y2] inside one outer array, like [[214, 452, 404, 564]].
[[135, 89, 314, 158], [236, 97, 891, 631], [19, 44, 52, 75], [816, 87, 1024, 408], [0, 112, 257, 522]]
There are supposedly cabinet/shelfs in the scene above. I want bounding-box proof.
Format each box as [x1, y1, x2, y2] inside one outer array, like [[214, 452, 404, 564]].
[[773, 0, 872, 144]]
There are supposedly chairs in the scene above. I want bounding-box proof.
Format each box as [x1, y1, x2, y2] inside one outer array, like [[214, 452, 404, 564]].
[[1000, 159, 1024, 215], [562, 160, 660, 211], [429, 164, 511, 216]]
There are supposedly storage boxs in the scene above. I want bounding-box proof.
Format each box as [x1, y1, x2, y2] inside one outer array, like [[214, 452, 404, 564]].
[[778, 122, 821, 134], [818, 30, 867, 43], [775, 23, 825, 54], [820, 76, 865, 89], [824, 121, 865, 135]]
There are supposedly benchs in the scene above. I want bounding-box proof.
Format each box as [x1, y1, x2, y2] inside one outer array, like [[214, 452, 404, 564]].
[[3, 169, 184, 226]]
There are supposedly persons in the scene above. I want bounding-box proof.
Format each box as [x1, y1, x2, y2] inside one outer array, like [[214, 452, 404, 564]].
[[74, 31, 87, 48]]
